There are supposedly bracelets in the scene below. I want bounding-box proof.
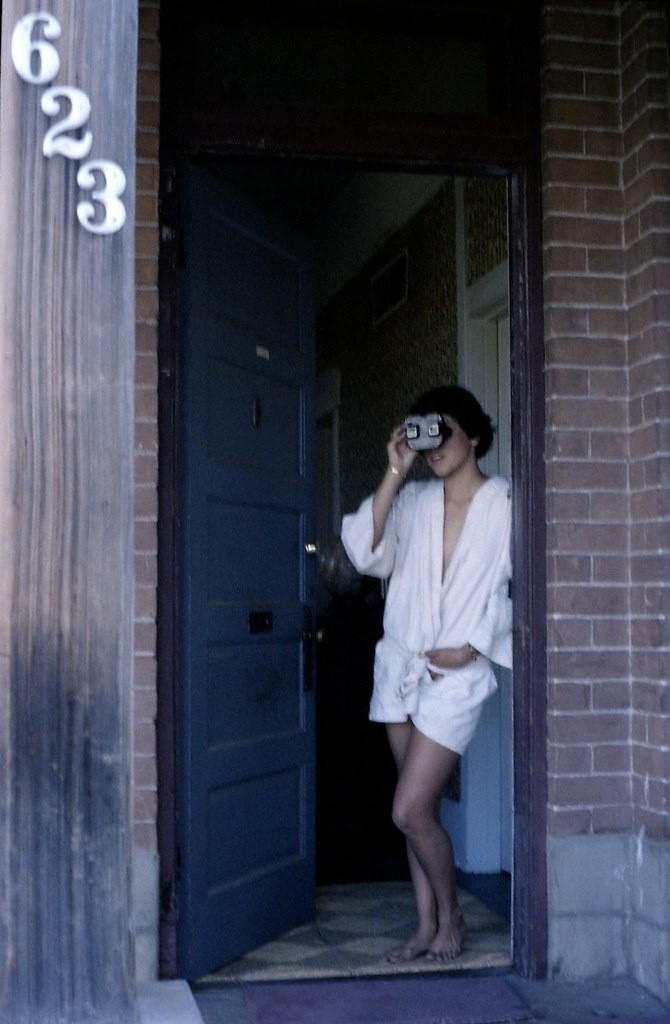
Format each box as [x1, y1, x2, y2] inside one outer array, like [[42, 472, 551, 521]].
[[467, 642, 481, 661], [389, 463, 406, 478]]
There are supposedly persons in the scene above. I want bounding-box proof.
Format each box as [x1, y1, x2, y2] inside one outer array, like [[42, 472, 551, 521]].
[[340, 383, 512, 965]]
